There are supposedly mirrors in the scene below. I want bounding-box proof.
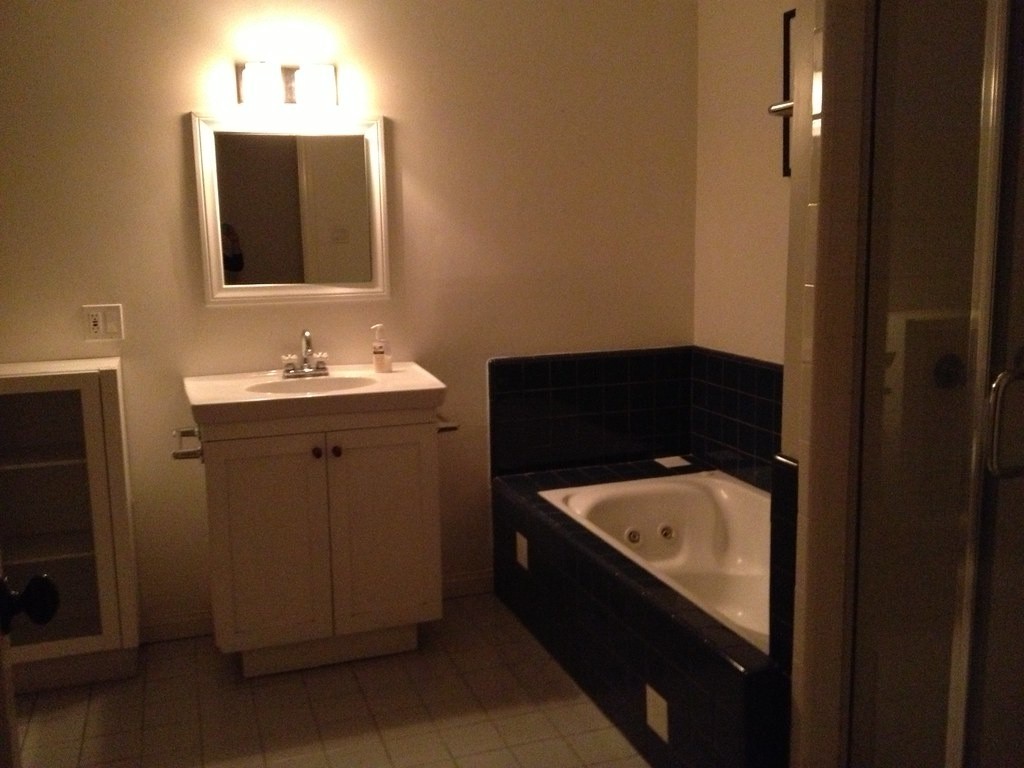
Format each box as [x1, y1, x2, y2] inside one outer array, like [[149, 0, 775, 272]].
[[188, 112, 389, 304]]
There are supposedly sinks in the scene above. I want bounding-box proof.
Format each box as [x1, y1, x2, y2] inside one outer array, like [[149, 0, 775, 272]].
[[244, 375, 378, 395]]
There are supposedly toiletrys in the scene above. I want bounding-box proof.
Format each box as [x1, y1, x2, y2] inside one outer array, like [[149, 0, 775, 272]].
[[369, 323, 394, 375]]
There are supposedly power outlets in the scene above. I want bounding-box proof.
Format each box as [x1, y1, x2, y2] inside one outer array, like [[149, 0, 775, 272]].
[[83, 304, 124, 341]]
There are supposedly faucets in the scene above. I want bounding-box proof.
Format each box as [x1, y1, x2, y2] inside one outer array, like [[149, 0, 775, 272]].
[[299, 329, 315, 378]]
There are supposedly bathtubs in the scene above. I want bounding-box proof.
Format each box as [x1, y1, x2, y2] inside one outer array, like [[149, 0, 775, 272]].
[[539, 469, 771, 658]]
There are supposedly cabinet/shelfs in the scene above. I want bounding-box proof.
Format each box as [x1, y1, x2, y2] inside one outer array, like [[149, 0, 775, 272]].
[[0, 358, 144, 691], [187, 359, 449, 678]]
